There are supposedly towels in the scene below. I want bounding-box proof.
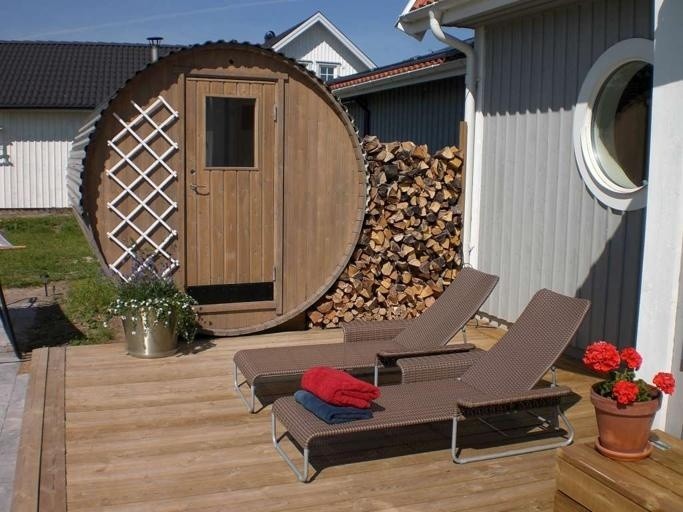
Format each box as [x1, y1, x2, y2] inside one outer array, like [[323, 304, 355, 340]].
[[293, 390, 373, 424], [293, 364, 382, 409]]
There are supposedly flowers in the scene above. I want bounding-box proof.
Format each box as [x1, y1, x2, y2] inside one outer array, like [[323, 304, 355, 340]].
[[581, 340, 676, 405], [98, 259, 199, 347]]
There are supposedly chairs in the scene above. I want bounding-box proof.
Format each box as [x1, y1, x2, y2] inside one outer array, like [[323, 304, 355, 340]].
[[234, 267, 500, 414], [270, 287, 591, 482]]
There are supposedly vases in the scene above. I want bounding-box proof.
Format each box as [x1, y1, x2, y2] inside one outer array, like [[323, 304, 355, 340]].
[[117, 306, 180, 358], [589, 379, 663, 460]]
